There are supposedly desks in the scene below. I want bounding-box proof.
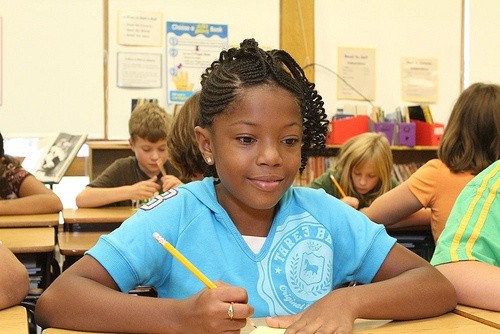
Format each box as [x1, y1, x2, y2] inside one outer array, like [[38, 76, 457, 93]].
[[1, 208, 500, 334]]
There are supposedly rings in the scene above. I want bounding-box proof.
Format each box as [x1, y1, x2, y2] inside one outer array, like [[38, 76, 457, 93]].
[[228, 302, 234, 319]]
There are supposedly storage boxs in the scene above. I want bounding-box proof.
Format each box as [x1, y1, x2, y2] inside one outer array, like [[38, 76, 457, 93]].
[[410, 120, 444, 146], [326, 115, 369, 145], [369, 122, 415, 146]]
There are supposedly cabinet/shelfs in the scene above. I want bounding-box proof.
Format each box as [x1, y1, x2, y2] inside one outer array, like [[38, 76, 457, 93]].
[[85, 140, 441, 182]]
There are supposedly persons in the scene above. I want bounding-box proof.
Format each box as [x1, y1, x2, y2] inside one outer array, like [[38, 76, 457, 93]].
[[33, 38, 458, 334], [309, 132, 400, 214], [166, 91, 219, 182], [429, 158, 500, 311], [367, 83, 500, 247], [0, 133, 63, 215], [0, 243, 29, 310], [76, 103, 192, 209]]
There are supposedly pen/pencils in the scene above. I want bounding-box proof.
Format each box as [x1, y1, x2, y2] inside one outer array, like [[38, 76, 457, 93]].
[[157, 161, 166, 178], [330, 175, 349, 199], [154, 233, 258, 329]]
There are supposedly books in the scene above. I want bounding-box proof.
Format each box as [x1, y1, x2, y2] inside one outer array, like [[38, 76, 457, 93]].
[[370, 104, 434, 124], [128, 285, 152, 293], [21, 262, 44, 298], [290, 156, 427, 188]]
